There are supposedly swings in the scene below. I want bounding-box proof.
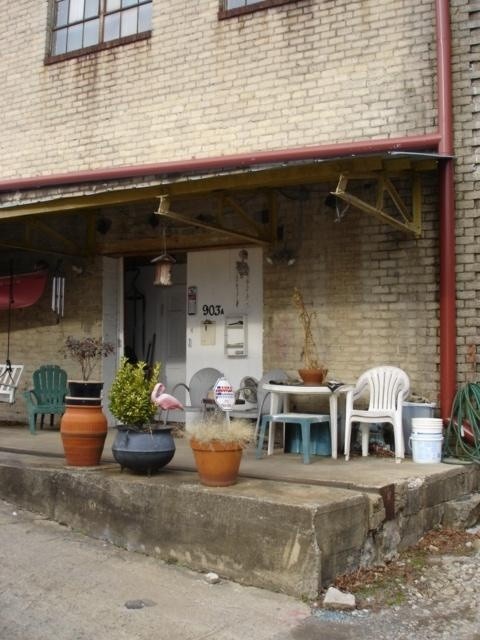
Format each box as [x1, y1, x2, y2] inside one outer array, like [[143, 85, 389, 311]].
[[0, 247, 24, 404]]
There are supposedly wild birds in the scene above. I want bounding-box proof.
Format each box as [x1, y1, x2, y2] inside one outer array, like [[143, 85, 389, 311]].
[[150, 382, 184, 427]]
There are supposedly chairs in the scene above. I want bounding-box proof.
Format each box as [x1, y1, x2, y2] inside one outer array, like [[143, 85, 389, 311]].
[[171, 364, 412, 463], [23, 361, 68, 436]]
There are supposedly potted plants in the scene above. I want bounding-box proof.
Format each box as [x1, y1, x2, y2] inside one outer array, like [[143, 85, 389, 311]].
[[289, 286, 331, 387], [54, 329, 123, 468], [172, 408, 269, 489], [107, 353, 177, 477]]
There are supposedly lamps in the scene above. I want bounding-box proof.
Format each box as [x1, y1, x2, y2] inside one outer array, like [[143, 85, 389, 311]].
[[149, 217, 179, 287]]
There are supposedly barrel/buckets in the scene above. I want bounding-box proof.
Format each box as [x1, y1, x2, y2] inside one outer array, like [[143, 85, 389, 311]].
[[393, 404, 433, 456], [408, 418, 444, 464]]
